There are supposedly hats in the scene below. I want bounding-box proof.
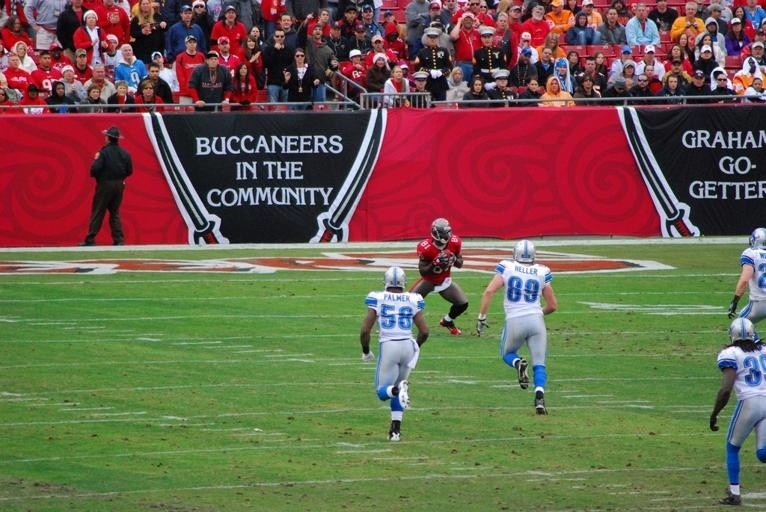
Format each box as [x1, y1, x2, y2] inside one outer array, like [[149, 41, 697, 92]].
[[383, 10, 393, 17], [362, 5, 373, 12], [106, 34, 119, 44], [399, 60, 408, 68], [218, 37, 230, 45], [83, 10, 98, 21], [101, 126, 125, 140], [413, 0, 766, 84], [356, 24, 365, 32], [60, 64, 74, 76], [371, 35, 383, 43], [373, 53, 387, 65], [224, 5, 236, 13], [50, 43, 61, 50], [185, 35, 197, 45], [76, 48, 87, 57], [151, 52, 162, 60], [331, 21, 340, 29], [350, 49, 361, 59], [181, 5, 192, 13]]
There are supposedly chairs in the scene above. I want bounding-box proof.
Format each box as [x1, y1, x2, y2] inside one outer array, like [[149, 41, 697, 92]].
[[375, 0, 766, 110], [158, 69, 327, 114]]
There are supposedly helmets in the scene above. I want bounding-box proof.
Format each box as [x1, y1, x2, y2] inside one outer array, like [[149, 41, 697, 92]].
[[513, 239, 536, 264], [750, 227, 766, 248], [729, 317, 756, 343], [431, 217, 452, 244], [383, 267, 407, 290]]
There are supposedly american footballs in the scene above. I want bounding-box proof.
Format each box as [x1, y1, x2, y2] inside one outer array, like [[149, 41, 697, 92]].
[[439, 249, 450, 269]]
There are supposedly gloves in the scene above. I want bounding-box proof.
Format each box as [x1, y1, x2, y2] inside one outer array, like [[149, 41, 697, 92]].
[[727, 294, 741, 319], [432, 249, 457, 268], [362, 352, 375, 363], [476, 318, 490, 337]]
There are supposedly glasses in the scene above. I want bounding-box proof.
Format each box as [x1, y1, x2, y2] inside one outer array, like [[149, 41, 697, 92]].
[[275, 35, 285, 39], [296, 54, 305, 58], [195, 5, 204, 8]]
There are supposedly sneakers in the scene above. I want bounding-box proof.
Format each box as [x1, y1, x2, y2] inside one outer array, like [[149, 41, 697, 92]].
[[386, 431, 402, 443], [440, 317, 462, 336], [78, 241, 96, 246], [112, 241, 125, 245], [517, 358, 530, 391], [534, 398, 548, 416], [398, 379, 411, 410], [719, 494, 743, 506]]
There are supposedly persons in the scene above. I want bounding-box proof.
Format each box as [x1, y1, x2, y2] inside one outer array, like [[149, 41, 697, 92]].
[[710, 317, 766, 504], [0, 0, 765, 113], [477, 240, 557, 415], [408, 218, 468, 336], [727, 227, 766, 323], [359, 267, 429, 440], [79, 126, 132, 246]]
